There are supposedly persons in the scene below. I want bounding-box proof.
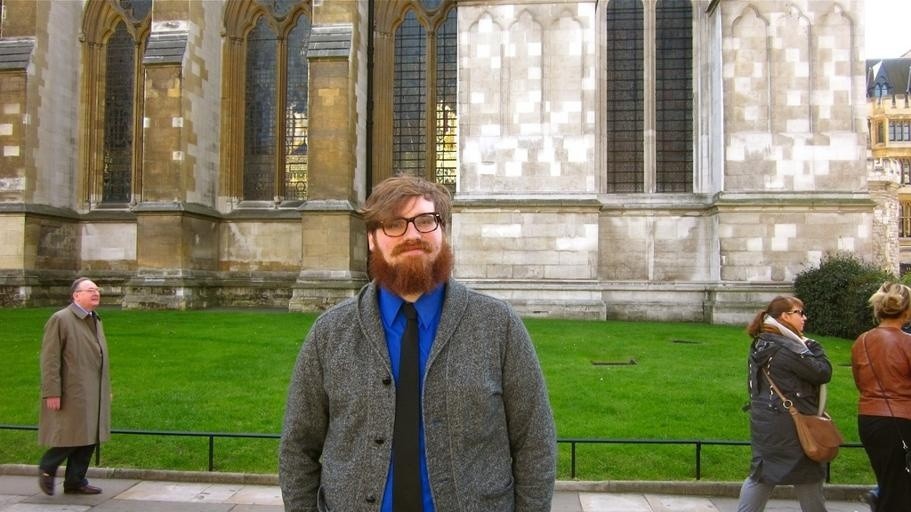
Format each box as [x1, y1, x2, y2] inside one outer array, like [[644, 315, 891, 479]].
[[279, 172, 556, 511], [38, 276, 110, 495], [737, 295, 832, 512], [850, 280, 911, 512]]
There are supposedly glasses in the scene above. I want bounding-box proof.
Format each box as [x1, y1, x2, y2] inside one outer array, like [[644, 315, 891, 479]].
[[371, 212, 447, 237], [786, 309, 809, 318]]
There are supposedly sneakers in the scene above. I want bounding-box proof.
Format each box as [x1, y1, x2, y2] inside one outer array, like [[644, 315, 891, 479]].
[[863, 486, 881, 512]]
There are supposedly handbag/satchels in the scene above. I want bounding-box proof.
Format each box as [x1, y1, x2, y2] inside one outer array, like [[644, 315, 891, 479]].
[[789, 404, 844, 462]]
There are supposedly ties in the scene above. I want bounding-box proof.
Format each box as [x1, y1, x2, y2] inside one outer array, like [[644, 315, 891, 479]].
[[393, 303, 424, 510]]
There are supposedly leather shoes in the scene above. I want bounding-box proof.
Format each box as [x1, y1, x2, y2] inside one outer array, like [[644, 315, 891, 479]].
[[63, 480, 102, 494], [38, 465, 55, 495]]
